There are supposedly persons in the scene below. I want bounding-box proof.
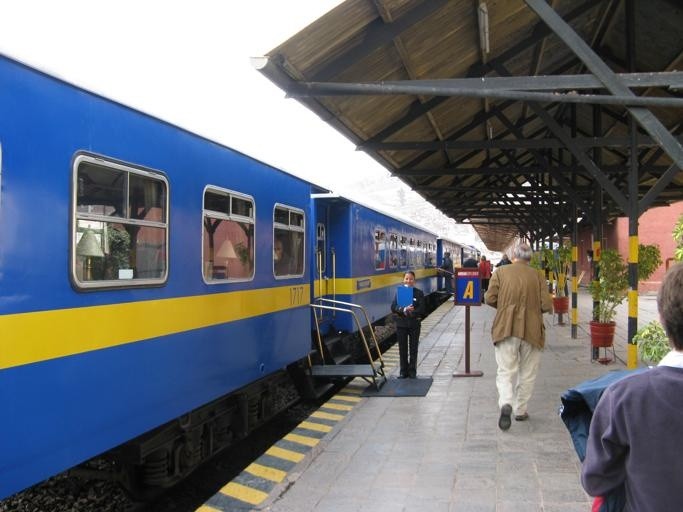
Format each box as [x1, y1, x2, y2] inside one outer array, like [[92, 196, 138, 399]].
[[463, 254, 477, 267], [580, 263, 682, 511], [482, 240, 552, 430], [479, 255, 490, 291], [390, 271, 426, 379], [442, 252, 454, 293], [494, 254, 512, 267], [273, 237, 290, 277]]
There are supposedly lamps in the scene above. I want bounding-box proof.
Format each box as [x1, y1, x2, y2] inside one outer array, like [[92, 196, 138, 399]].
[[216, 239, 237, 279], [76, 224, 107, 281]]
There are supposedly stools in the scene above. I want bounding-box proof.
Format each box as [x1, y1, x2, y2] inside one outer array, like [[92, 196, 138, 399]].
[[552, 312, 570, 327], [590, 345, 616, 363]]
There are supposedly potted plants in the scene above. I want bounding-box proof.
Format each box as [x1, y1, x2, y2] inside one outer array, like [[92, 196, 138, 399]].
[[589, 242, 664, 347], [541, 240, 573, 314]]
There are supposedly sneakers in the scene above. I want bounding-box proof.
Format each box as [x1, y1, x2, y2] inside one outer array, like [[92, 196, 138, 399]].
[[499, 404, 512, 431], [397, 374, 416, 378], [515, 412, 528, 421]]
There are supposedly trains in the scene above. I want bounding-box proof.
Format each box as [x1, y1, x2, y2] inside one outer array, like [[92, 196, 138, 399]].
[[0, 55, 484, 506]]
